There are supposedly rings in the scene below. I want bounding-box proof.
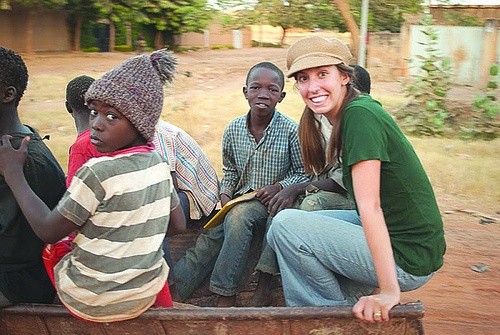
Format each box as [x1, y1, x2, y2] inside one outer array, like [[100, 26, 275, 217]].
[[374, 311, 381, 320]]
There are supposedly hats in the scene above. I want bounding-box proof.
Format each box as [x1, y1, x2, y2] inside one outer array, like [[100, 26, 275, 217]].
[[286, 36, 352, 77], [84, 48, 178, 141]]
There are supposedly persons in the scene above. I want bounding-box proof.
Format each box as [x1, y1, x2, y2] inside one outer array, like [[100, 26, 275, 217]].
[[0, 48, 186, 324], [65, 77, 96, 189], [241, 65, 371, 307], [152, 119, 221, 267], [173, 61, 313, 308], [0, 46, 68, 309], [266, 34, 447, 323]]
